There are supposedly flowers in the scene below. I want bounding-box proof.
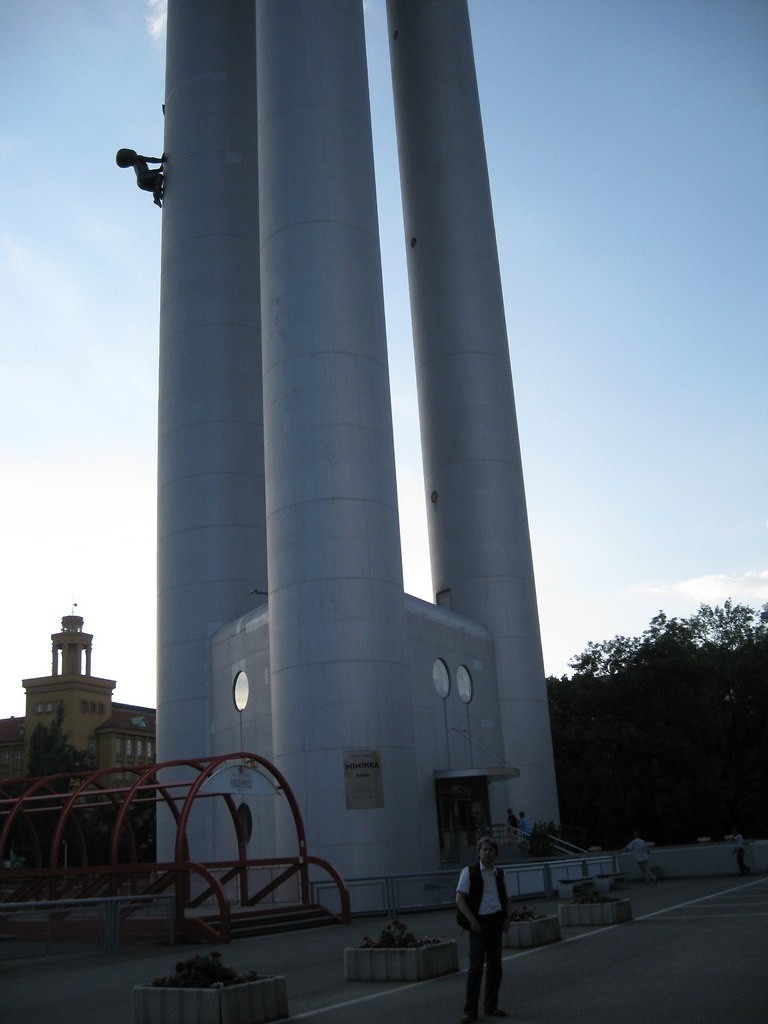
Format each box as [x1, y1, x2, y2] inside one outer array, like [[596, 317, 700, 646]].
[[510, 905, 548, 921], [141, 949, 260, 989], [567, 891, 623, 904], [356, 916, 441, 948]]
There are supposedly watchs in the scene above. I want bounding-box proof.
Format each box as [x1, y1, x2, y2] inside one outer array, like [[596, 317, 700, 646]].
[[507, 914, 513, 921]]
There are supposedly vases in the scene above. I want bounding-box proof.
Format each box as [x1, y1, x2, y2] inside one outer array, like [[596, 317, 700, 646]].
[[501, 913, 562, 948], [342, 939, 461, 983], [130, 973, 290, 1024], [559, 897, 633, 927]]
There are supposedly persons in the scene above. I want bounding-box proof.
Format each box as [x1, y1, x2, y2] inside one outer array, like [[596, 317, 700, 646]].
[[731, 828, 751, 875], [518, 811, 531, 843], [456, 837, 512, 1023], [506, 809, 519, 841], [618, 832, 658, 887]]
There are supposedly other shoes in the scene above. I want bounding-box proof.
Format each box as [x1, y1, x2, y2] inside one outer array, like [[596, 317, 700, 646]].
[[462, 1013, 478, 1023], [738, 868, 751, 876], [485, 1008, 507, 1017], [654, 875, 658, 886], [645, 884, 650, 887]]
